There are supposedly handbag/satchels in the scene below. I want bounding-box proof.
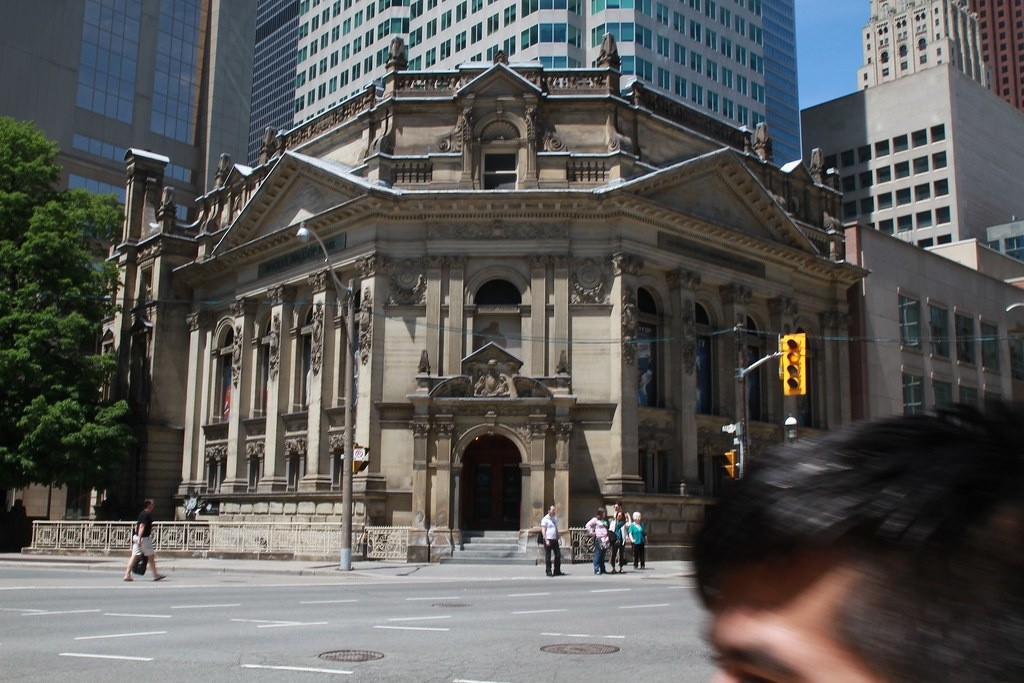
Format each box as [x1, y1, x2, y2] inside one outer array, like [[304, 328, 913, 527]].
[[608, 530, 617, 545], [598, 536, 611, 550], [133, 553, 147, 575], [537, 532, 544, 544]]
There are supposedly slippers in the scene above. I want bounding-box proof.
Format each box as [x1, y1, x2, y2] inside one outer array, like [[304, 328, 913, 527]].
[[153, 574, 166, 581], [123, 577, 133, 581]]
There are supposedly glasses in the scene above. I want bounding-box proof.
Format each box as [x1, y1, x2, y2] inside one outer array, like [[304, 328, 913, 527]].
[[617, 515, 622, 517], [614, 506, 618, 509]]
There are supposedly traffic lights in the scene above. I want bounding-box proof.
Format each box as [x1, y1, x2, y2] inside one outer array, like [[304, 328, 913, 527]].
[[725, 450, 735, 481], [783, 333, 806, 395]]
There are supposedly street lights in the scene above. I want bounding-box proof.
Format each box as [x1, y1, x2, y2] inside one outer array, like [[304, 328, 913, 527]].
[[297, 220, 360, 572]]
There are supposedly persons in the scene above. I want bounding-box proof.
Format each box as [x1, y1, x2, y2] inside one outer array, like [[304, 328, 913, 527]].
[[628, 511, 649, 570], [689, 395, 1024, 683], [122, 497, 167, 583], [608, 511, 631, 574], [613, 501, 632, 566], [585, 507, 612, 575], [540, 505, 566, 577]]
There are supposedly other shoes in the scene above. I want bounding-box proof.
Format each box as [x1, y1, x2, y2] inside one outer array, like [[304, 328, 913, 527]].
[[554, 571, 564, 576], [546, 571, 553, 576], [594, 570, 600, 575], [619, 570, 625, 573], [634, 565, 638, 570], [601, 570, 608, 574], [641, 565, 645, 570], [612, 570, 616, 573]]
